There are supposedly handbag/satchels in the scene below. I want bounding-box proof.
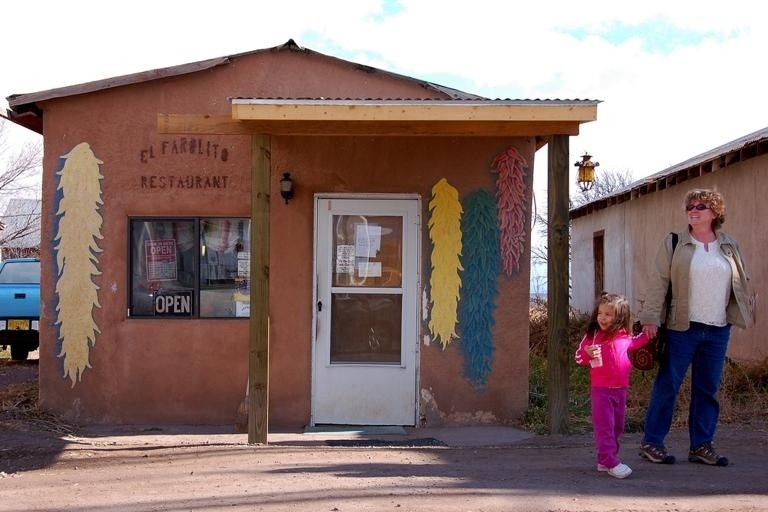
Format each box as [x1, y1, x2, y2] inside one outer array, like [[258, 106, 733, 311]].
[[629, 321, 666, 371]]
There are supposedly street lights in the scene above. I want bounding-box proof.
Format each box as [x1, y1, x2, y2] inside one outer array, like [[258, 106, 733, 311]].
[[575, 150, 600, 191]]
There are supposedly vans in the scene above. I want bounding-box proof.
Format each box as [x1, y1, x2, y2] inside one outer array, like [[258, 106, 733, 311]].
[[0, 257, 41, 360]]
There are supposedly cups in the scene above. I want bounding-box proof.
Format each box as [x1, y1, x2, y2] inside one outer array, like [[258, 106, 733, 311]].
[[588, 344, 603, 368]]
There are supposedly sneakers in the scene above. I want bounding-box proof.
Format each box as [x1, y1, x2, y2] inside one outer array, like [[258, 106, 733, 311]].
[[690, 444, 728, 466], [598, 463, 633, 478], [638, 443, 676, 464]]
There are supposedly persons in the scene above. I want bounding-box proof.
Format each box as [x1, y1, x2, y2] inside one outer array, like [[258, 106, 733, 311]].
[[573, 289, 656, 478], [640, 188, 755, 466]]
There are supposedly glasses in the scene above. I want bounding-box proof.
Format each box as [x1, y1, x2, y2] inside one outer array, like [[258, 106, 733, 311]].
[[686, 202, 710, 212]]
